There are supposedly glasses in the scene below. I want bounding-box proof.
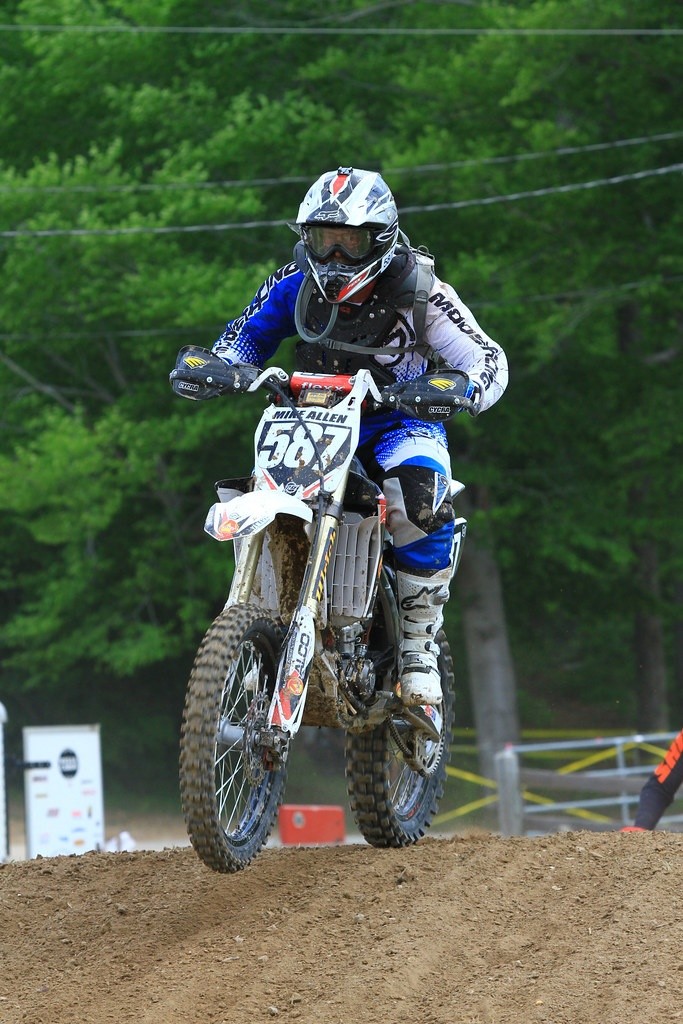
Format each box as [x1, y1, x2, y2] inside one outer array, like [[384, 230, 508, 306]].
[[298, 217, 398, 266]]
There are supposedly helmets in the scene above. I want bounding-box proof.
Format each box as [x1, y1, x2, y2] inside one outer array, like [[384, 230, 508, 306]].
[[296, 167, 399, 303]]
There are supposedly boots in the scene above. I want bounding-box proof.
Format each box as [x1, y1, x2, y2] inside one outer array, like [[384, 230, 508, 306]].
[[392, 565, 458, 706]]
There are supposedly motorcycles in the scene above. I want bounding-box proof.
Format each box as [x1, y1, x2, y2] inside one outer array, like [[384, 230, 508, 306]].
[[163, 344, 470, 877]]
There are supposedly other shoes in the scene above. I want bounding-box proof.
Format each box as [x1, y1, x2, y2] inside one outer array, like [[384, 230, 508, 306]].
[[620, 825, 646, 832]]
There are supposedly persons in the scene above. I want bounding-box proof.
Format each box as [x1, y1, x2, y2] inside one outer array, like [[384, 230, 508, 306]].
[[210, 167, 510, 706]]
